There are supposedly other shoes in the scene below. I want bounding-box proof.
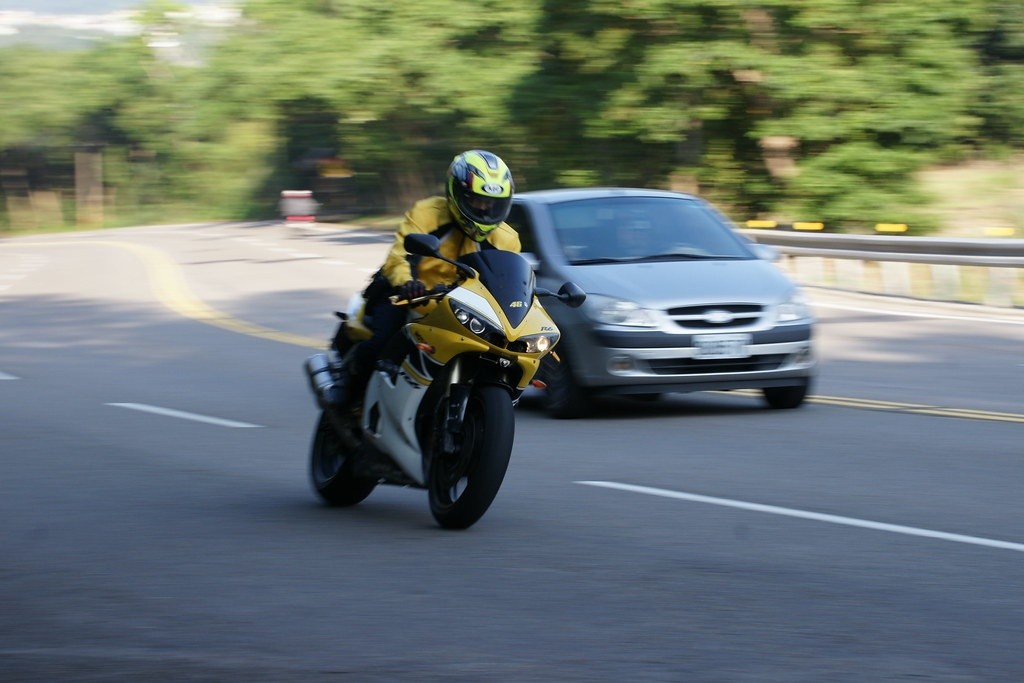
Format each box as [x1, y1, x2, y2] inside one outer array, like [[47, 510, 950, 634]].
[[334, 396, 363, 429]]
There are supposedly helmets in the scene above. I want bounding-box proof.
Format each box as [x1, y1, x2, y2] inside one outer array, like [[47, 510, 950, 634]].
[[446, 149, 516, 243]]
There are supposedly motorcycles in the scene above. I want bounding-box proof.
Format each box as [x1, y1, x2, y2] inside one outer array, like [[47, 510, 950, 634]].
[[303, 233, 587, 531]]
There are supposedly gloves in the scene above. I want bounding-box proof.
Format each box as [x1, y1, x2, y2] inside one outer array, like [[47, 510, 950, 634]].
[[395, 280, 425, 308]]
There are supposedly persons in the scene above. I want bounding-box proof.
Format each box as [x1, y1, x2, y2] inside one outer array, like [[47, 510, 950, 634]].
[[325, 150, 521, 452]]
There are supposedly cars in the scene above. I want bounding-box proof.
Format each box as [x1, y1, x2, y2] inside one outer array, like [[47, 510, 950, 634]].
[[510, 187, 819, 416]]
